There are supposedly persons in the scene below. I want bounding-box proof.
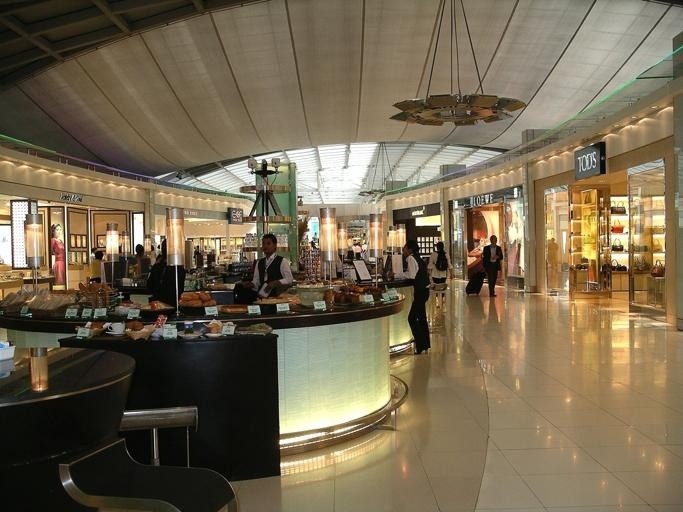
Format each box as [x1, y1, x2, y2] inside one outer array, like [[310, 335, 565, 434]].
[[243, 234, 294, 299], [388, 240, 431, 354], [483, 235, 503, 297], [50, 224, 66, 285], [427, 242, 452, 311], [548, 238, 558, 264], [89, 240, 199, 308], [352, 242, 368, 259]]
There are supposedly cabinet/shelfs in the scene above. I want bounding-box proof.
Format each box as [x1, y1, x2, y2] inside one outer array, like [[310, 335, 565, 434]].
[[572, 189, 628, 298]]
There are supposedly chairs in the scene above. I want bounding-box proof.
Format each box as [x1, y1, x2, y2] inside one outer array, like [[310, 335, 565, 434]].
[[119, 406, 199, 466], [57, 437, 238, 512]]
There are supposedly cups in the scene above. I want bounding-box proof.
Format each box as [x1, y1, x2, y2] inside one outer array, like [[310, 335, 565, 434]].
[[30, 346, 48, 393], [108, 323, 125, 333]]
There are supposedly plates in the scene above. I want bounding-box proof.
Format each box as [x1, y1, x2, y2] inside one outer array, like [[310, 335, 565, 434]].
[[178, 330, 222, 339], [106, 332, 126, 336]]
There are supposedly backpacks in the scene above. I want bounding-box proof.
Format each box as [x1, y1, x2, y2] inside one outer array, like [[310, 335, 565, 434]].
[[433, 250, 448, 271]]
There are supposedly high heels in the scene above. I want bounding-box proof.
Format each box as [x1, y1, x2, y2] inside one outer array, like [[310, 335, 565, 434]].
[[414, 344, 428, 354]]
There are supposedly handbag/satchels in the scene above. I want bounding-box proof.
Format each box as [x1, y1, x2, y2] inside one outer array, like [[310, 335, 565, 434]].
[[652, 239, 662, 251], [612, 238, 623, 251], [611, 201, 626, 214], [611, 259, 627, 271], [633, 256, 649, 271], [611, 218, 623, 233], [651, 259, 664, 277]]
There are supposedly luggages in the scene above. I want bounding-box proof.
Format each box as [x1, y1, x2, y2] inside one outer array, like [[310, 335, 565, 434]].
[[466, 272, 486, 296]]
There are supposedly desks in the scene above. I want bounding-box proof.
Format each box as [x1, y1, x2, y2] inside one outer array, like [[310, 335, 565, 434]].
[[0, 346, 136, 512], [57, 331, 281, 481]]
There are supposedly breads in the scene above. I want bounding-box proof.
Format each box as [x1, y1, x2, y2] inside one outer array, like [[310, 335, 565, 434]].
[[178, 292, 216, 307], [221, 305, 247, 312]]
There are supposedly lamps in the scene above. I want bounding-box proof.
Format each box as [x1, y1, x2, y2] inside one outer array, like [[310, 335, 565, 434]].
[[358, 143, 394, 197], [389, 0, 527, 127]]
